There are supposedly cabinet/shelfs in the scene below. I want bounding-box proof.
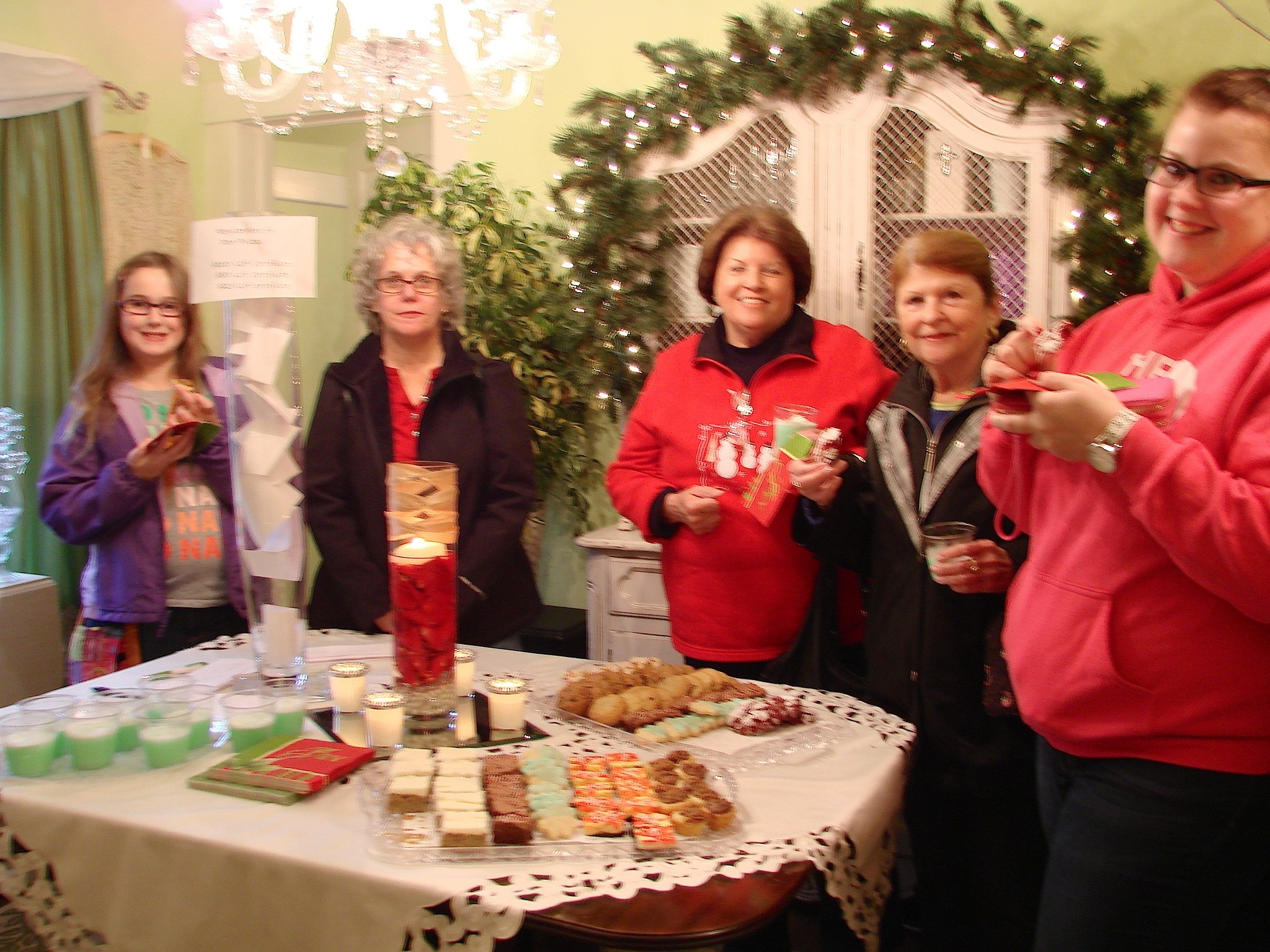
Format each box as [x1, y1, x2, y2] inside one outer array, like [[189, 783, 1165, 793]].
[[618, 53, 1123, 360], [574, 522, 683, 664]]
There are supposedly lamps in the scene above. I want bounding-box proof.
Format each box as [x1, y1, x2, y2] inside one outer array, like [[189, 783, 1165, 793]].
[[177, 0, 562, 175]]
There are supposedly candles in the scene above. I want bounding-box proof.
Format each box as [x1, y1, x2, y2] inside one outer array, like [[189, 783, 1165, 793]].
[[454, 647, 477, 696], [390, 536, 447, 566], [362, 692, 404, 747], [486, 677, 528, 730], [328, 661, 368, 713]]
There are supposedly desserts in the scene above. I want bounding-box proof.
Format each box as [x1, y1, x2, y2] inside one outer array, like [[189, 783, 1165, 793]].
[[567, 749, 735, 853]]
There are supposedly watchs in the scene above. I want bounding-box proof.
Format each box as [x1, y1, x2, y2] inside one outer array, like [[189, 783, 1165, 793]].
[[1085, 408, 1139, 473]]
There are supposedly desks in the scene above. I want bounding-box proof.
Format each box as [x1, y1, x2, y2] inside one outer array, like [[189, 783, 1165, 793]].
[[0, 628, 915, 952]]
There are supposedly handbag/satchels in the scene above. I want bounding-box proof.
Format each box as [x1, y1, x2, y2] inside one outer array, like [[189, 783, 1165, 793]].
[[983, 609, 1023, 715], [762, 559, 876, 698], [67, 608, 140, 681]]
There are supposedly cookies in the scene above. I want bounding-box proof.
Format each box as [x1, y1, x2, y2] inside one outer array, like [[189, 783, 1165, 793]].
[[171, 379, 195, 416], [556, 656, 815, 744], [1034, 320, 1073, 363], [517, 743, 582, 839], [814, 426, 841, 468]]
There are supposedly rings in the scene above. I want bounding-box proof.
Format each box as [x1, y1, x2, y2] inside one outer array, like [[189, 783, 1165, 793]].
[[970, 560, 980, 572], [988, 343, 999, 357]]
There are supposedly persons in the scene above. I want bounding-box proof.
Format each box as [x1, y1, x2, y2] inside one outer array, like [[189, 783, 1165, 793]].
[[786, 230, 1042, 952], [36, 251, 271, 685], [304, 218, 543, 652], [978, 68, 1270, 952], [606, 207, 900, 681]]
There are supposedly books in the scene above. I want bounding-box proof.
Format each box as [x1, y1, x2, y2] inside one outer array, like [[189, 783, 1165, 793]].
[[187, 734, 376, 804]]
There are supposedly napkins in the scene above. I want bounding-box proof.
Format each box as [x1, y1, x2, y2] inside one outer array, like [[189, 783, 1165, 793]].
[[260, 604, 299, 666]]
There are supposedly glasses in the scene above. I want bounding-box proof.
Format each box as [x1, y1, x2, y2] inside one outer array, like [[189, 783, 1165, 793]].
[[1142, 155, 1270, 198], [373, 274, 447, 294], [117, 298, 190, 318]]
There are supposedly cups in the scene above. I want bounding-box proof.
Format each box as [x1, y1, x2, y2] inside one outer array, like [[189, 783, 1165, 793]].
[[361, 691, 406, 750], [327, 663, 370, 713], [160, 684, 216, 748], [0, 710, 63, 777], [919, 521, 978, 580], [485, 677, 527, 729], [15, 694, 78, 759], [88, 687, 155, 752], [139, 674, 191, 692], [218, 690, 275, 753], [385, 462, 460, 715], [772, 404, 819, 449], [55, 703, 127, 769], [250, 620, 305, 688], [454, 648, 477, 696], [134, 702, 194, 769], [262, 678, 311, 736]]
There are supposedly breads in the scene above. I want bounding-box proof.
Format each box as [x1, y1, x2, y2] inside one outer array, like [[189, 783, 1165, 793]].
[[385, 748, 534, 849]]
